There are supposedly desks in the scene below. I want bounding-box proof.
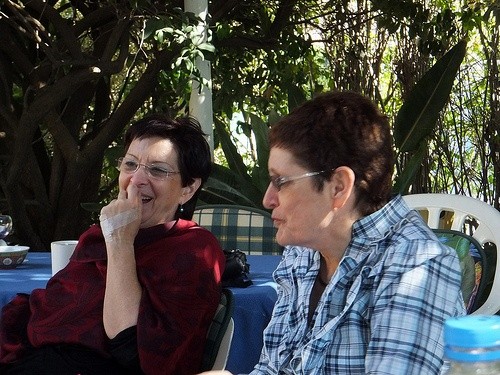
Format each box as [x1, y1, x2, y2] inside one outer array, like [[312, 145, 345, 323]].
[[0, 251, 285, 375]]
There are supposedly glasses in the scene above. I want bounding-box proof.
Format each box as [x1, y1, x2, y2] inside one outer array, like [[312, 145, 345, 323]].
[[269, 167, 336, 192], [117, 156, 179, 182]]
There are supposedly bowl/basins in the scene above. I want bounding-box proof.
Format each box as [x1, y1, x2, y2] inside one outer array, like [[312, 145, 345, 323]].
[[0, 245, 30, 270]]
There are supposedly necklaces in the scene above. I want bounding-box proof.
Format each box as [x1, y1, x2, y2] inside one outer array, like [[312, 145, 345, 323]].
[[317, 275, 327, 286]]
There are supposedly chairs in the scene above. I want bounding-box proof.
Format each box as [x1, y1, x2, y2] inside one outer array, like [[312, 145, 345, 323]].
[[198, 285, 237, 375], [190, 204, 283, 255], [400, 192, 500, 318]]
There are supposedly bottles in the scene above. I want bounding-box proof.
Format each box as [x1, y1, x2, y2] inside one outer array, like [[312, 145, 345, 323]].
[[439, 313, 500, 375]]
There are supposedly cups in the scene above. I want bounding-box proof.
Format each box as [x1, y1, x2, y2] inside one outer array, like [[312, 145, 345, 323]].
[[0, 214, 13, 239], [50, 240, 80, 278]]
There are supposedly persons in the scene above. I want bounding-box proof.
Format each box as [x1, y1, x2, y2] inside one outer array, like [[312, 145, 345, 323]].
[[196, 90, 468, 375], [0, 113, 228, 375]]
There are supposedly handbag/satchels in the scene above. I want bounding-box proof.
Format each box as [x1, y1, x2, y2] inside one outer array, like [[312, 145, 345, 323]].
[[220, 249, 250, 284]]
